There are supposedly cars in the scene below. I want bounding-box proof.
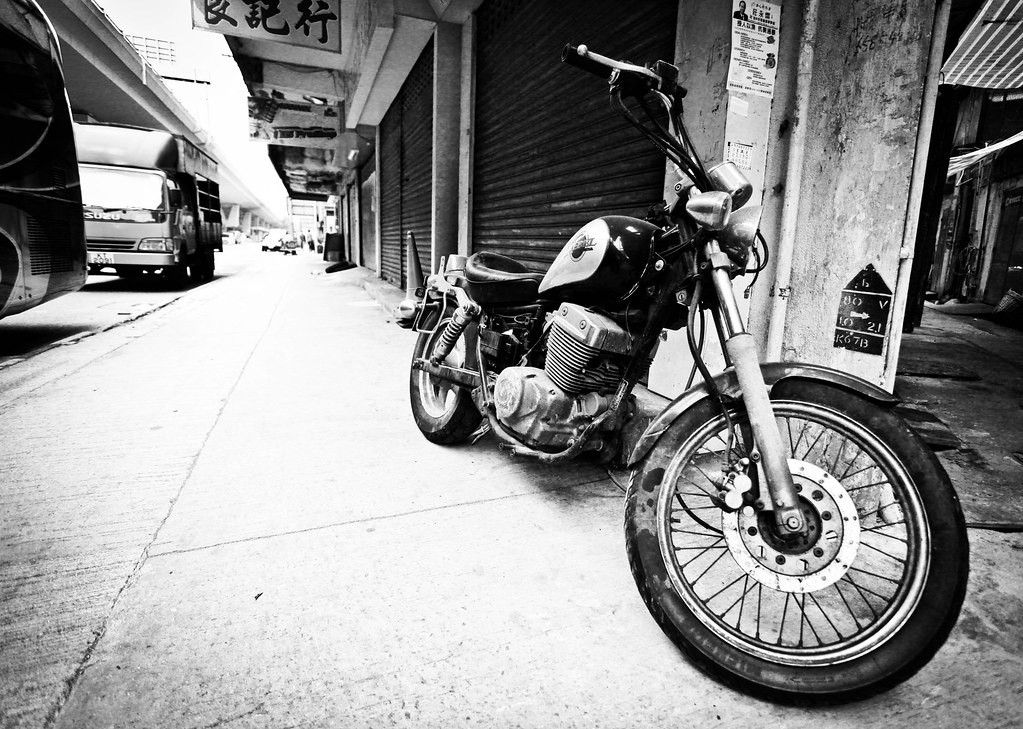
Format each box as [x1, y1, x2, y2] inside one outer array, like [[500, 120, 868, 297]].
[[261, 229, 291, 252], [0, 0, 91, 317]]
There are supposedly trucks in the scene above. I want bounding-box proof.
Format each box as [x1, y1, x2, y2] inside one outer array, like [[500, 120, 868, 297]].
[[73, 119, 223, 292]]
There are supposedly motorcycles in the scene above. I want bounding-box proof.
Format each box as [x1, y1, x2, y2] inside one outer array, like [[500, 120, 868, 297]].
[[408, 44, 972, 711]]
